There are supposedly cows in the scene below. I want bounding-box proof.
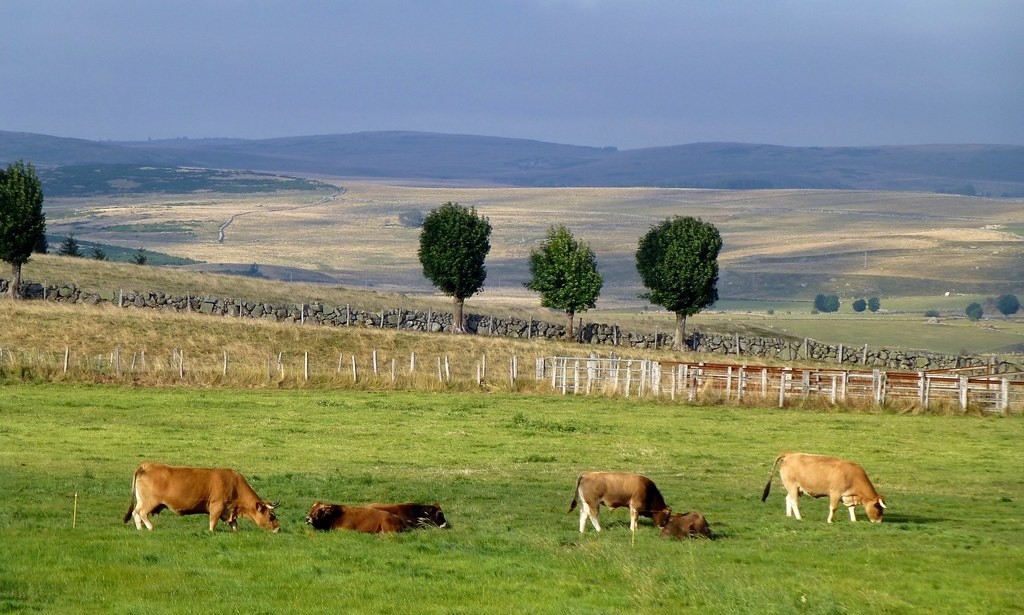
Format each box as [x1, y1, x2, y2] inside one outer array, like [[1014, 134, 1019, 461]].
[[566, 471, 714, 541], [123, 461, 282, 534], [761, 451, 888, 523], [305, 501, 448, 532]]
